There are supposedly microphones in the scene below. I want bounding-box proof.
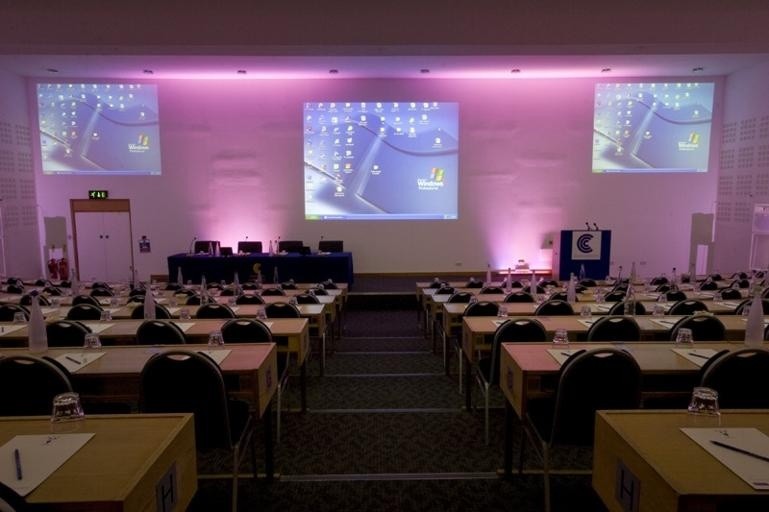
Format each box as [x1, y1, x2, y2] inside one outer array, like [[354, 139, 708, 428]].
[[593, 222, 598, 231], [129, 265, 147, 291], [585, 222, 591, 231], [245, 236, 248, 255], [189, 236, 197, 255], [319, 235, 324, 254], [673, 266, 679, 289], [618, 264, 623, 283], [277, 236, 280, 255]]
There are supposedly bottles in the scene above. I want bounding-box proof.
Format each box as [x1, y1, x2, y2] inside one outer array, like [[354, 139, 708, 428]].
[[208, 242, 213, 256], [268, 239, 278, 256], [29, 296, 49, 354], [743, 296, 765, 347], [68, 265, 280, 322], [484, 261, 700, 317], [215, 242, 220, 257]]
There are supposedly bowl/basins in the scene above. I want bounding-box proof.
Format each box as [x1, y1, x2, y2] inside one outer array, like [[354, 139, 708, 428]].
[[279, 253, 288, 256]]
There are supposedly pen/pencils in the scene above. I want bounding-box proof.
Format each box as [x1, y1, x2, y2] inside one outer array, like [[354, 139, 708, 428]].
[[561, 353, 571, 356], [15, 450, 22, 479], [709, 440, 769, 462], [496, 322, 502, 324], [660, 321, 675, 324], [66, 356, 80, 364], [689, 353, 710, 359], [585, 321, 594, 323]]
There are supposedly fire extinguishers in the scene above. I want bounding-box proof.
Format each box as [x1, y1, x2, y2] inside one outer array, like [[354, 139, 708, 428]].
[[47, 258, 69, 280]]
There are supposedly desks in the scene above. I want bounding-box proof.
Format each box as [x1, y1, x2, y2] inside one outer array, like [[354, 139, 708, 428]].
[[0, 411, 195, 511], [590, 408, 769, 512]]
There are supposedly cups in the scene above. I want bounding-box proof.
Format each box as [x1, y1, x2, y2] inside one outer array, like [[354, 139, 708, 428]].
[[552, 326, 569, 347], [713, 292, 724, 305], [50, 392, 86, 433], [686, 387, 722, 419], [0, 278, 333, 326], [208, 329, 224, 348], [676, 328, 695, 351], [83, 333, 102, 352]]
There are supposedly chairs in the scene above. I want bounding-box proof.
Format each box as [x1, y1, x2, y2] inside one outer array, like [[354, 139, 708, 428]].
[[518, 348, 643, 509], [137, 351, 259, 511], [0, 277, 348, 477], [166, 237, 354, 282], [415, 269, 768, 447]]
